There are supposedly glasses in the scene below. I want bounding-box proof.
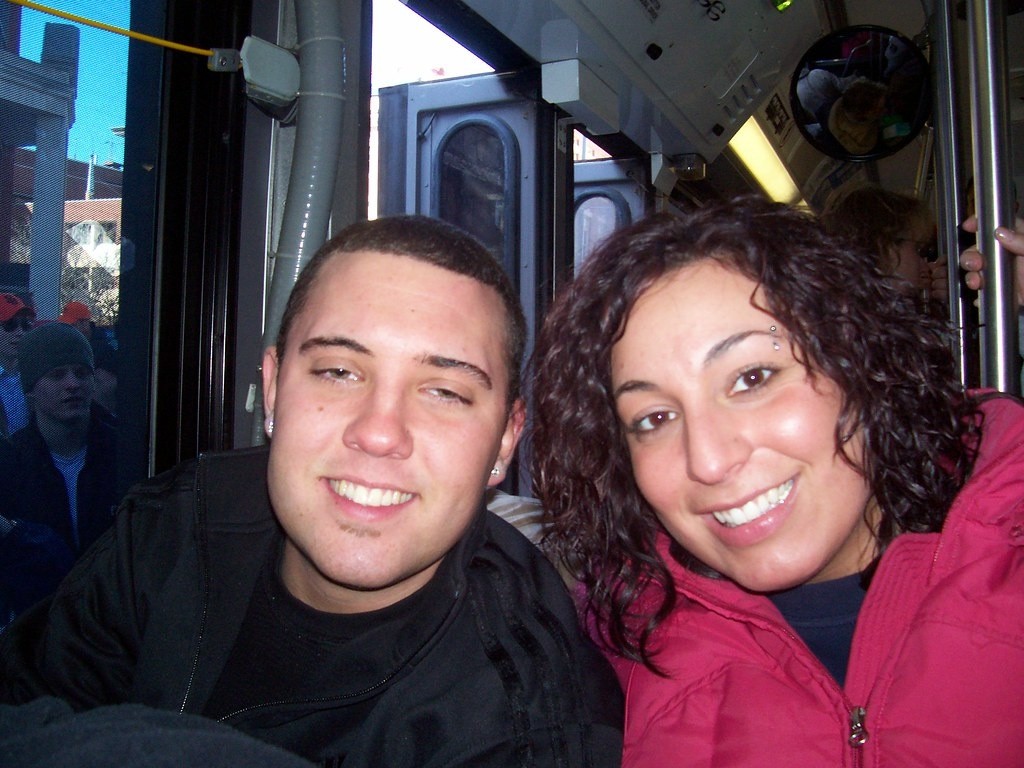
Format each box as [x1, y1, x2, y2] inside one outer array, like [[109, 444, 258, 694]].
[[894, 238, 931, 259], [0, 317, 33, 333]]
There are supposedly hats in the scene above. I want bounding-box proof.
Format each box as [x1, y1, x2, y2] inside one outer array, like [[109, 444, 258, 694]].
[[19, 321, 94, 394], [797, 69, 843, 113], [0, 293, 36, 322], [57, 302, 90, 324]]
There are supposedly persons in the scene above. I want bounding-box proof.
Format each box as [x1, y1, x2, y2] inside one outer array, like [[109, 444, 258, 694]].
[[792, 26, 931, 161], [0, 186, 1024, 768]]
